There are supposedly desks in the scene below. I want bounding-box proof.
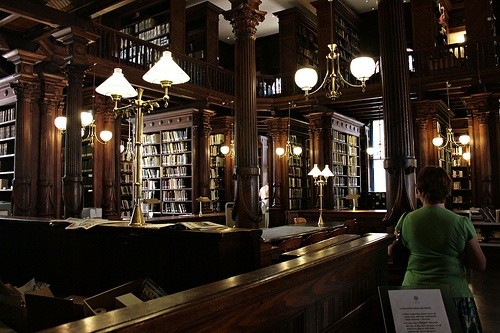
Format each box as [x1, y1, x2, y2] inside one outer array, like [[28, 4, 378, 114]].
[[287, 210, 387, 233]]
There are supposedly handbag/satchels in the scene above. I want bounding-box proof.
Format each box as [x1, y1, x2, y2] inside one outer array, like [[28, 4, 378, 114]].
[[389, 209, 410, 267]]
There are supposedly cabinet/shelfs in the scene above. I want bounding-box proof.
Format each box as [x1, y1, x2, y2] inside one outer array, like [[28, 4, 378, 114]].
[[0, 0, 365, 219], [413, 95, 500, 246]]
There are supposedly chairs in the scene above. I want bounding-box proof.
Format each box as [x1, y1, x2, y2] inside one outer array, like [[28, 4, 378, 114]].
[[280, 220, 357, 251]]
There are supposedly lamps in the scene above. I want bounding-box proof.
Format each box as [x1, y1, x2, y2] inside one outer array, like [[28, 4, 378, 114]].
[[294, 0, 377, 100], [307, 164, 335, 226], [82, 63, 114, 146], [220, 101, 235, 157], [94, 50, 192, 225], [276, 101, 303, 158], [432, 79, 472, 150]]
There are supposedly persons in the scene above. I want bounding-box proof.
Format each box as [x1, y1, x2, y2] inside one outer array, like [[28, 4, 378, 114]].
[[388, 166, 486, 333]]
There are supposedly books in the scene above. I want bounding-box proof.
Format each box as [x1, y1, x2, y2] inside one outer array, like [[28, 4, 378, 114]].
[[437, 122, 471, 203], [0, 108, 16, 189], [141, 130, 187, 214], [289, 136, 311, 210], [332, 130, 357, 208], [451, 207, 500, 242], [210, 134, 224, 210]]
